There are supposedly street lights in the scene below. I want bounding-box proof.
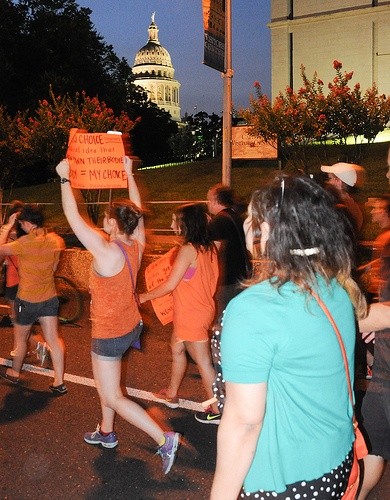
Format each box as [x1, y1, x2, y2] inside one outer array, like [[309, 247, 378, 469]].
[[193, 104, 198, 113]]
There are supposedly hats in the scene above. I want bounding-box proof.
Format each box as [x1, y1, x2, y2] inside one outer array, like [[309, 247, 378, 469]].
[[321, 162, 357, 187]]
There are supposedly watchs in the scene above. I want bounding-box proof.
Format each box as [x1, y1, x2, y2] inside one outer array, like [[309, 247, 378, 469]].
[[59, 178, 71, 184]]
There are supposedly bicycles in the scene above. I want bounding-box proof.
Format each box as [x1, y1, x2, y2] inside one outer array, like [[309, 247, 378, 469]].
[[0, 262, 83, 324]]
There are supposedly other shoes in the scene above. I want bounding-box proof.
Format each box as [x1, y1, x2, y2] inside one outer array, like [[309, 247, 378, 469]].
[[0, 366, 20, 384], [49, 382, 68, 393]]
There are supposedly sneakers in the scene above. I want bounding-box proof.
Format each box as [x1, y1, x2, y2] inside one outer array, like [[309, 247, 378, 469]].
[[195, 406, 222, 424], [153, 432, 182, 474], [152, 390, 179, 408], [84, 423, 118, 448], [31, 341, 49, 367]]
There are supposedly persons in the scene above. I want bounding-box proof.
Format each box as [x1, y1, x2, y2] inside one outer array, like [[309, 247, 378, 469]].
[[0, 200, 68, 394], [206, 162, 390, 499], [55, 154, 182, 476], [137, 199, 224, 427]]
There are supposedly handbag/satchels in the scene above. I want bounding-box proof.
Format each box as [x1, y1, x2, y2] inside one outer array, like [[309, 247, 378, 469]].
[[340, 422, 368, 500], [129, 321, 143, 350]]
